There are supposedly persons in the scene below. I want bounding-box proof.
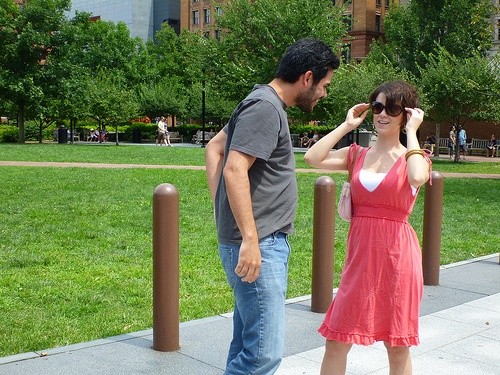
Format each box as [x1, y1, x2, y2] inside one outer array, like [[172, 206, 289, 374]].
[[486, 134, 497, 157], [86, 126, 109, 143], [457, 124, 467, 160], [448, 126, 456, 160], [205, 38, 340, 375], [303, 80, 434, 375], [192, 128, 218, 145], [156, 116, 172, 147], [424, 132, 436, 155], [301, 129, 320, 148]]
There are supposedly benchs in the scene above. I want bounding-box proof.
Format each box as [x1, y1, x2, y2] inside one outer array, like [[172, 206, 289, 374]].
[[168, 132, 181, 142], [192, 132, 216, 145], [438, 138, 450, 154], [467, 138, 500, 158]]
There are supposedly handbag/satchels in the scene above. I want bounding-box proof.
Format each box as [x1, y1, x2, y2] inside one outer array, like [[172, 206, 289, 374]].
[[338, 147, 365, 221], [487, 143, 493, 149], [448, 140, 453, 148]]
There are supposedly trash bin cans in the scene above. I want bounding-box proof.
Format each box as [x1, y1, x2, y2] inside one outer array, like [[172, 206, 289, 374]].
[[336, 130, 372, 151], [58, 128, 67, 143]]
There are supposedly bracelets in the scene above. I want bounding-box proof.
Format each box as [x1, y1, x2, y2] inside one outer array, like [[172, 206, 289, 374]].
[[405, 149, 425, 161]]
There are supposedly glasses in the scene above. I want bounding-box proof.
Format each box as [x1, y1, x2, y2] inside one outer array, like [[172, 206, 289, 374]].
[[372, 101, 410, 117]]
